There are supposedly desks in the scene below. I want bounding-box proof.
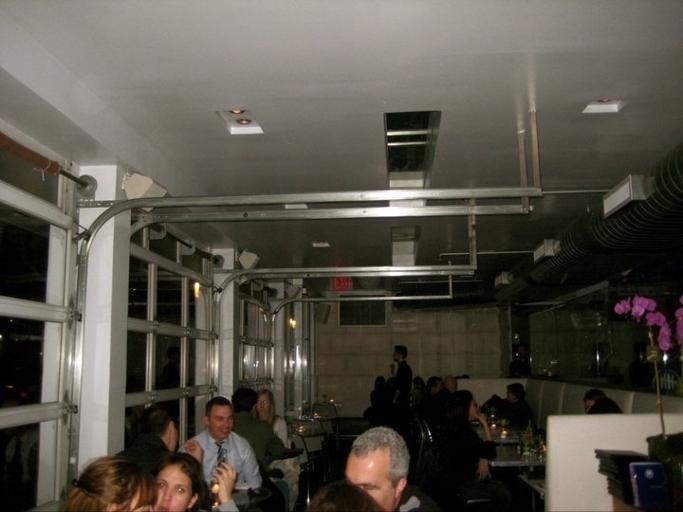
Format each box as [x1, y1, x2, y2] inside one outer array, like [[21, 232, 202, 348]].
[[469, 417, 544, 510]]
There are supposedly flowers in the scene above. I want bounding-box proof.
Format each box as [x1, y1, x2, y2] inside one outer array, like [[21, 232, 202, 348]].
[[616, 294, 682, 440]]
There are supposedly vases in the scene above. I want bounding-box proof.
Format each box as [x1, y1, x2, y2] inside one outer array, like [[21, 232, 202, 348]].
[[644, 432, 683, 510]]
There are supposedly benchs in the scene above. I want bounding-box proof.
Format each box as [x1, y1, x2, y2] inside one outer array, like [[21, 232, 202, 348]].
[[452, 373, 683, 511]]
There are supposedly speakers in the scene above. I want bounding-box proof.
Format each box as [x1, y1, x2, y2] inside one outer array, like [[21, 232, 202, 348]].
[[121, 173, 166, 212], [285, 285, 300, 299], [314, 303, 331, 325], [239, 250, 261, 272]]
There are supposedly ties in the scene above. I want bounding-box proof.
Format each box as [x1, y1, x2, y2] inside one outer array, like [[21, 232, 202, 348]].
[[215, 441, 225, 466]]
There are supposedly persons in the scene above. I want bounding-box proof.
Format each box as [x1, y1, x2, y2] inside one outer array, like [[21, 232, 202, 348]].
[[307, 427, 442, 512], [365, 343, 537, 480], [583, 389, 623, 413], [59, 388, 289, 512]]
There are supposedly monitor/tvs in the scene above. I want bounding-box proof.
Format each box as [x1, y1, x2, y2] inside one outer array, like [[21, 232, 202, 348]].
[[338, 291, 388, 328]]
